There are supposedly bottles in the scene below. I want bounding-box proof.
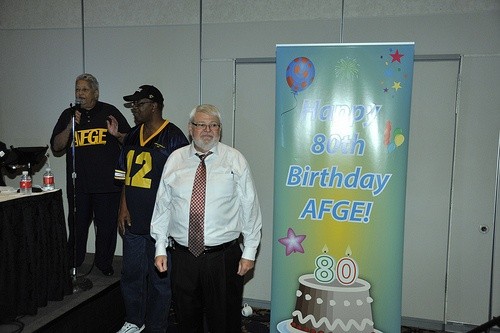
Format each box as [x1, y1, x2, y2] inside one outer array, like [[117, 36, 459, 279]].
[[42, 168, 55, 191], [20, 170, 32, 195]]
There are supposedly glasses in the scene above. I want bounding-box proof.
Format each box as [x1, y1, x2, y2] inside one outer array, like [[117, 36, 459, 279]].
[[191, 122, 220, 131], [131, 101, 154, 108]]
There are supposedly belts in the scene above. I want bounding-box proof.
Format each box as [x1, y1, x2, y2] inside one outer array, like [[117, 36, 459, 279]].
[[173, 239, 238, 255]]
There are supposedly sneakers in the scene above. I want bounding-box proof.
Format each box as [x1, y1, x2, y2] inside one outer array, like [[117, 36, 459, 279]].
[[116, 322, 145, 333]]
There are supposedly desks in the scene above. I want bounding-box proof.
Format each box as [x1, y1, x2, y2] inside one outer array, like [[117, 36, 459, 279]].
[[0, 186, 74, 321]]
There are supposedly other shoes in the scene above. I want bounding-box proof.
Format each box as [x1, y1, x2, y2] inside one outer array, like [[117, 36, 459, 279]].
[[98, 263, 114, 276]]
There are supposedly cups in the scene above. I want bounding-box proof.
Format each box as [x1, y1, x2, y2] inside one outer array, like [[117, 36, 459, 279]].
[[241, 303, 253, 317]]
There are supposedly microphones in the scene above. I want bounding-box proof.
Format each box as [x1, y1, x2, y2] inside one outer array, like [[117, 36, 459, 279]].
[[75, 99, 81, 112]]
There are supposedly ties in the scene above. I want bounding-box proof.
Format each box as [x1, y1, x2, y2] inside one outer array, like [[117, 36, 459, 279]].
[[188, 152, 213, 258]]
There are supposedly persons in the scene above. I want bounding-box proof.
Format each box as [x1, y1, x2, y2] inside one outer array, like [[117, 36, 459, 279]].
[[151, 104, 263, 333], [49, 73, 132, 275], [115, 85, 190, 333]]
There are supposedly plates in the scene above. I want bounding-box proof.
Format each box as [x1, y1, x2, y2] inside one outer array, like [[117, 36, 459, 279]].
[[0, 186, 17, 192]]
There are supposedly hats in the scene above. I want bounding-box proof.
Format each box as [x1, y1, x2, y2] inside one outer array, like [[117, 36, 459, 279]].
[[123, 85, 164, 102]]
